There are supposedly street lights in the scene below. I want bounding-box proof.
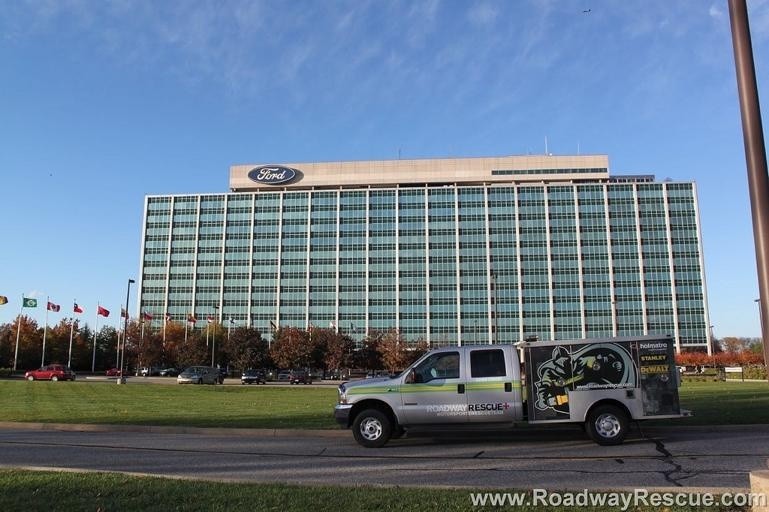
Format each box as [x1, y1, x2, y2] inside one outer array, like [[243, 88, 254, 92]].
[[120, 278, 135, 379]]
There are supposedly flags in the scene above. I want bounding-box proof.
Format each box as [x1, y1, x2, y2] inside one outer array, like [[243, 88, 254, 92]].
[[229, 316, 236, 324], [142, 310, 153, 320], [208, 313, 214, 323], [97, 305, 110, 318], [350, 322, 359, 333], [120, 307, 128, 318], [271, 320, 276, 328], [47, 301, 61, 312], [23, 297, 37, 307], [0, 295, 9, 305], [73, 303, 85, 314], [187, 313, 197, 322], [329, 321, 337, 329]]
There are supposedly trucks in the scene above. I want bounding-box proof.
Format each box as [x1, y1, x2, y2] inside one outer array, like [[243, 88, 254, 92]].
[[334, 334, 694, 448]]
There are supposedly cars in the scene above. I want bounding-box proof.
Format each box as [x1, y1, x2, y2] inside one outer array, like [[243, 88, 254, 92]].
[[24, 364, 76, 381], [106, 366, 404, 384]]
